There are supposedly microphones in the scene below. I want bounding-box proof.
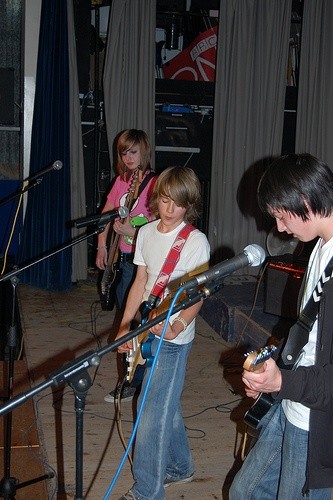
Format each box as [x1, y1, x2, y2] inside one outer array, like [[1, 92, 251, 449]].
[[75, 206, 129, 228], [183, 243, 265, 288], [26, 160, 63, 181]]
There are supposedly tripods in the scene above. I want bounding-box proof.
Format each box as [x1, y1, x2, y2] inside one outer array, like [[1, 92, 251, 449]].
[[0, 225, 106, 500]]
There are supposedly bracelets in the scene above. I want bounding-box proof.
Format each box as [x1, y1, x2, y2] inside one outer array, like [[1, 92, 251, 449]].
[[172, 318, 187, 332], [97, 246, 107, 250]]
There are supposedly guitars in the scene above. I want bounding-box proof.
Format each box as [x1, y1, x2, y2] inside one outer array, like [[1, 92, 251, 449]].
[[97, 164, 143, 312], [119, 272, 207, 398], [241, 336, 287, 439]]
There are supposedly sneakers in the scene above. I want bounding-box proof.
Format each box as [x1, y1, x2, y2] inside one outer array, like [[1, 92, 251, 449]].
[[164, 471, 194, 488], [103, 385, 137, 403], [117, 490, 164, 500]]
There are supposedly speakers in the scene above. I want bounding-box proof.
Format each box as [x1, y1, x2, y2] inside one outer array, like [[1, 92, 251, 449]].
[[264, 258, 307, 321]]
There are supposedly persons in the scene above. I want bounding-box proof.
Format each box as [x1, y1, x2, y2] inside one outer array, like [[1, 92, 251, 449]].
[[229, 153, 333, 500], [114, 167, 211, 500], [97, 130, 159, 403]]
